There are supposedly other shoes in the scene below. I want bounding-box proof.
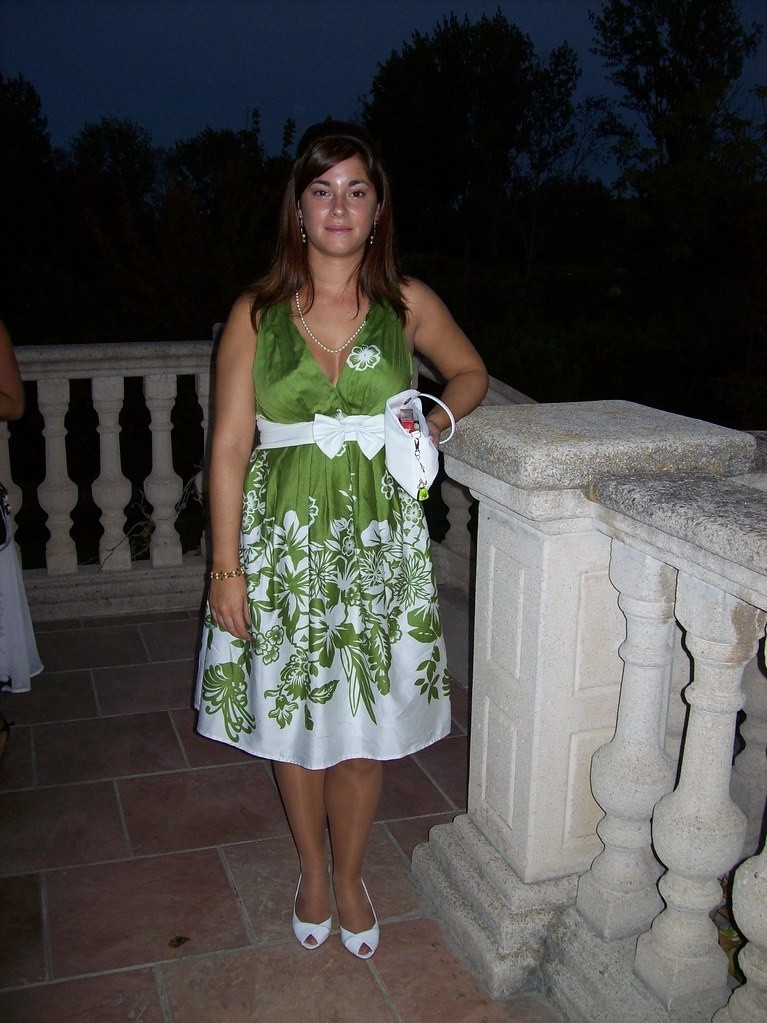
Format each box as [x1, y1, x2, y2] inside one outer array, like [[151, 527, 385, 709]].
[[340, 878, 380, 959], [293, 864, 333, 948]]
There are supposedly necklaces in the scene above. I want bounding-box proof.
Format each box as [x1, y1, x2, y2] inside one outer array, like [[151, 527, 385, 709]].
[[295, 290, 370, 353]]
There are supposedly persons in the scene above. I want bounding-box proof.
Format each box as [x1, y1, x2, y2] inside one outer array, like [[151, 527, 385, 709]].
[[0, 325, 44, 695], [194, 133, 488, 959]]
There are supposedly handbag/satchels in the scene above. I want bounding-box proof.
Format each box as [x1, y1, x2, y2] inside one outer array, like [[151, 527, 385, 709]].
[[384, 389, 455, 501]]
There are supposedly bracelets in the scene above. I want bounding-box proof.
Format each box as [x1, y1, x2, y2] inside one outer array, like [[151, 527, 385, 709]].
[[210, 569, 243, 581]]
